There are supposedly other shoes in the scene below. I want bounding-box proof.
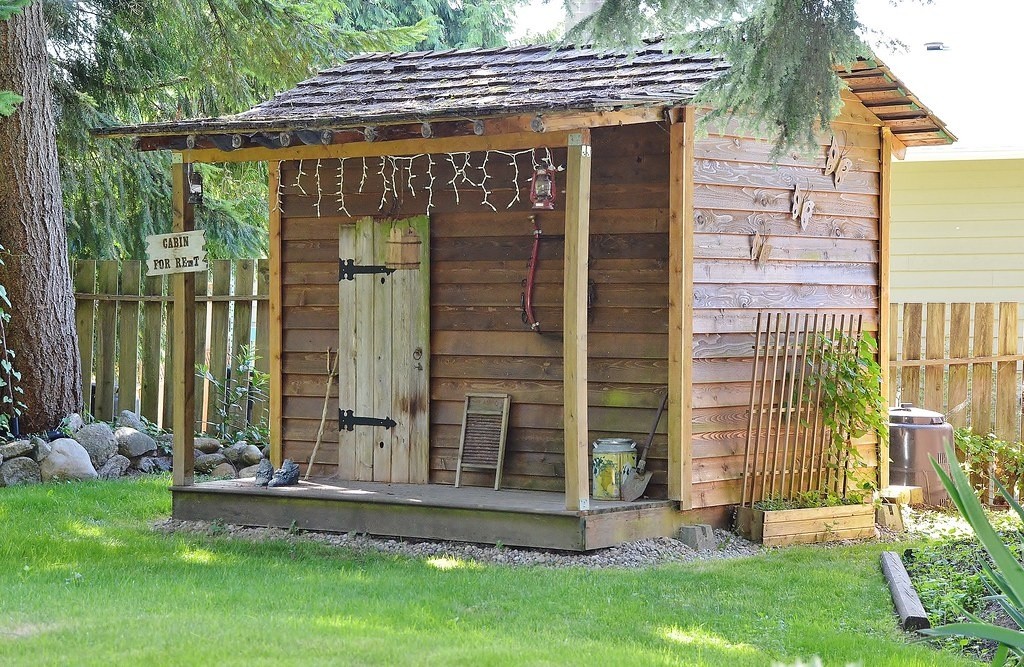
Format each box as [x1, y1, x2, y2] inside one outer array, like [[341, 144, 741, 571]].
[[254, 459, 273, 486], [268, 458, 300, 488]]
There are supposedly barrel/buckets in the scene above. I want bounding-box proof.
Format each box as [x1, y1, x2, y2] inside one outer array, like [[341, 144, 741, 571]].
[[592, 436, 637, 501], [889, 404, 954, 503]]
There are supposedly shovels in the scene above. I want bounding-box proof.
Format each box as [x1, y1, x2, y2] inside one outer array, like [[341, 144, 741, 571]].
[[622, 387, 668, 502]]
